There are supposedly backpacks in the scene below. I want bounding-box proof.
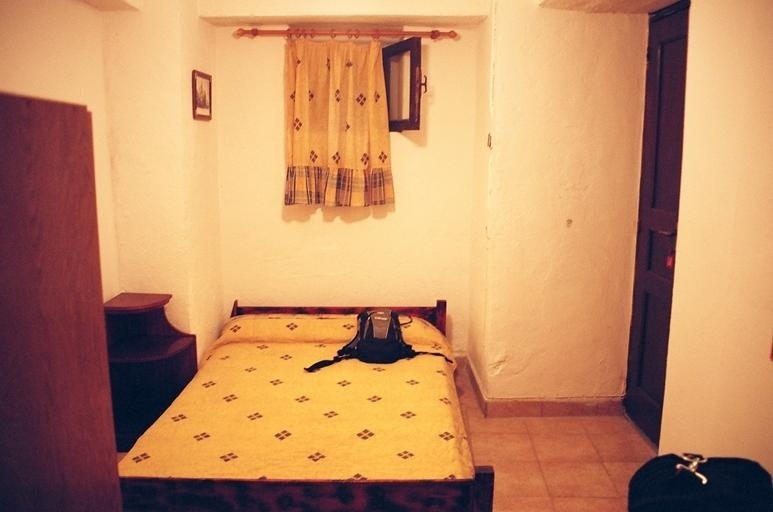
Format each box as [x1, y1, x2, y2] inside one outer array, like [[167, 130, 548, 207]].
[[338, 309, 414, 363]]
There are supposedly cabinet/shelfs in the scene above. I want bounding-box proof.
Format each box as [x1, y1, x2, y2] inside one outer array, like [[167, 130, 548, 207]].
[[101, 292, 197, 454]]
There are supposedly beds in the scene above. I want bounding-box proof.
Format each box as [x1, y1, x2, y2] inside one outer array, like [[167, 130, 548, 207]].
[[112, 297, 496, 512]]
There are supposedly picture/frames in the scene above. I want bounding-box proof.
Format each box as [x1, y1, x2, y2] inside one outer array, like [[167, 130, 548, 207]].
[[190, 69, 213, 122]]
